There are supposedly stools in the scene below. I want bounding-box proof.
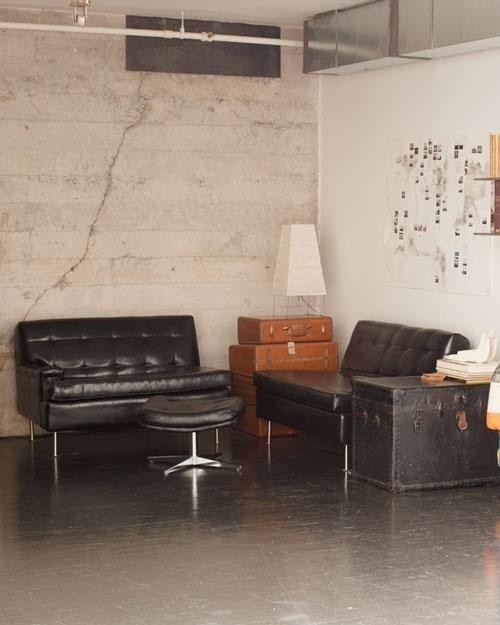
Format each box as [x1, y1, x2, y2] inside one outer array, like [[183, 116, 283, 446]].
[[140, 395, 247, 477]]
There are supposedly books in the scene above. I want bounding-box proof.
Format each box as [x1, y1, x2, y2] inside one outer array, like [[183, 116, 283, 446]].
[[436, 358, 500, 381]]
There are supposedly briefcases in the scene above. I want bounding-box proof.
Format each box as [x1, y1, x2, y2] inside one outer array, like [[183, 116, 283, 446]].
[[226, 341, 341, 439], [236, 314, 334, 346]]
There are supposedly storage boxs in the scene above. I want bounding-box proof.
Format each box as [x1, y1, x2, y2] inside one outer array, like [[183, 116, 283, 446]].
[[229, 341, 339, 444], [351, 376, 500, 494]]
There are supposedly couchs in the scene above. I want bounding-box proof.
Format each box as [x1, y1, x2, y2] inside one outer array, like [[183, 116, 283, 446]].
[[14, 315, 233, 457], [253, 320, 469, 472]]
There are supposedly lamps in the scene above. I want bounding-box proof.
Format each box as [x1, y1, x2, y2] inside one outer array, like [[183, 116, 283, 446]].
[[272, 224, 326, 318]]
